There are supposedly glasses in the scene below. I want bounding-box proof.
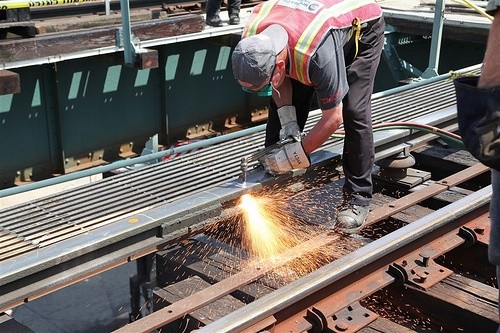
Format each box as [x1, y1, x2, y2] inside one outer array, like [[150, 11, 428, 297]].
[[240, 65, 277, 93]]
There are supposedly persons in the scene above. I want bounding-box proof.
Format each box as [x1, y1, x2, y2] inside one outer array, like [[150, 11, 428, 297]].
[[206, 0, 241, 26], [452, 0, 500, 333], [231, 0, 385, 233]]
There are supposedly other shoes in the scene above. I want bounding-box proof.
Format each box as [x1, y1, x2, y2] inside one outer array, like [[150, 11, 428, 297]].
[[230, 13, 240, 24], [206, 17, 223, 27]]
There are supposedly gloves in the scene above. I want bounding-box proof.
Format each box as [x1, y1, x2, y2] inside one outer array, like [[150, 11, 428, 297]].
[[276, 104, 301, 141], [453, 76, 500, 171], [257, 140, 312, 176]]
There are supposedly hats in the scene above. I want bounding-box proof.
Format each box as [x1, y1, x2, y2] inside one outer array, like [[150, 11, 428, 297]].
[[231, 24, 288, 89]]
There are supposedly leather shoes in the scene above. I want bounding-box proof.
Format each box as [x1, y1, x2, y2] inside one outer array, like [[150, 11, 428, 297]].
[[335, 202, 373, 233]]
